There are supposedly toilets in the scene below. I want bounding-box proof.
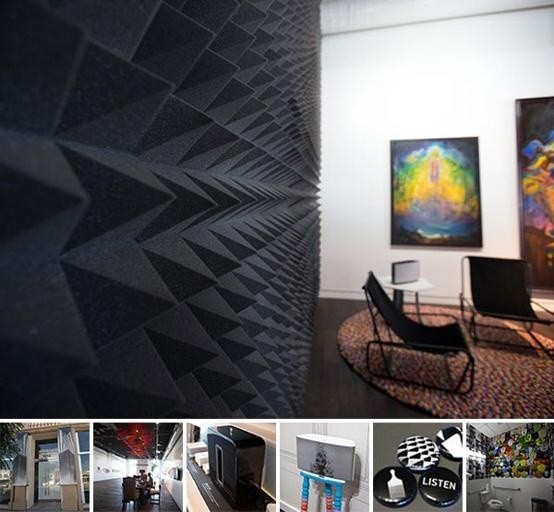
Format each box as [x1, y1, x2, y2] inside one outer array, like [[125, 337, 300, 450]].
[[480, 491, 504, 512]]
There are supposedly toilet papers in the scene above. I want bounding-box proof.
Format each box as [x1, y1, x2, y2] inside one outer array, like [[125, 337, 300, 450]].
[[507, 498, 511, 505]]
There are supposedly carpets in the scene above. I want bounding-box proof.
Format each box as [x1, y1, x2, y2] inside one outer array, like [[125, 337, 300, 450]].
[[338, 305, 554, 418]]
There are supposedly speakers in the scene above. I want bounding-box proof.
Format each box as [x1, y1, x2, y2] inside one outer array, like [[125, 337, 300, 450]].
[[296, 433, 355, 482]]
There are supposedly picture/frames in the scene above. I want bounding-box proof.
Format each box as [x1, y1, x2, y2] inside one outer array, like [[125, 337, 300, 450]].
[[390, 136, 483, 247], [515, 95, 554, 300]]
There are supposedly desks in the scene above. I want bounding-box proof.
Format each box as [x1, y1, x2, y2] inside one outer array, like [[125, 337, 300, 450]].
[[379, 275, 432, 326]]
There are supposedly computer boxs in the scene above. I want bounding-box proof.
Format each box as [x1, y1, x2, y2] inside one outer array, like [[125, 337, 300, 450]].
[[208, 425, 265, 508]]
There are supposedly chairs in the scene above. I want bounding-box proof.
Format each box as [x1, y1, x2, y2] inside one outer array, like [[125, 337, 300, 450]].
[[459, 256, 553, 359], [362, 271, 475, 397], [122, 476, 161, 510]]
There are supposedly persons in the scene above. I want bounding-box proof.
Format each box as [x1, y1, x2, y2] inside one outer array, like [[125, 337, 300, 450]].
[[144, 472, 153, 494]]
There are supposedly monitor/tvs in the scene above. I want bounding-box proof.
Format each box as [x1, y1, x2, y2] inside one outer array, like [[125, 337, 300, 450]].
[[174, 468, 180, 480]]
